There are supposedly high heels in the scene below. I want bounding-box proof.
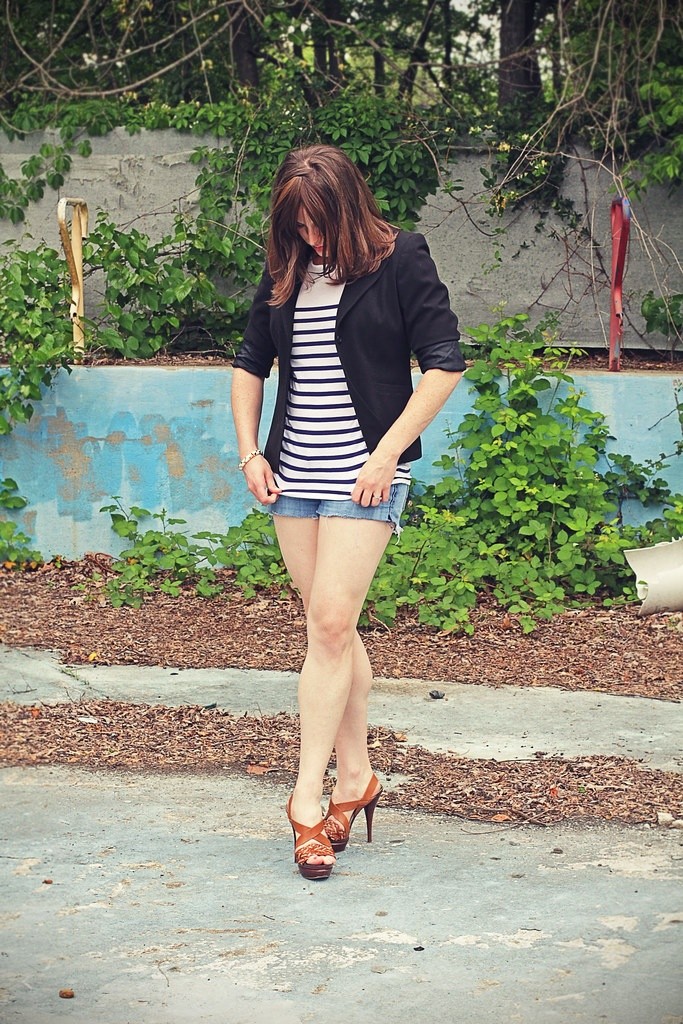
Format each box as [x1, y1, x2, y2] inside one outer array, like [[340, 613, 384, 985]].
[[323, 772, 383, 853], [286, 794, 335, 880]]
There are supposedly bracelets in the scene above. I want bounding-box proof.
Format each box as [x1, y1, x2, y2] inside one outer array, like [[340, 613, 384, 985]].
[[238, 450, 260, 471]]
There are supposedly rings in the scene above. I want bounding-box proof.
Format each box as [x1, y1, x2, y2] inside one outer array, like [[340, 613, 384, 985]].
[[376, 497, 382, 501]]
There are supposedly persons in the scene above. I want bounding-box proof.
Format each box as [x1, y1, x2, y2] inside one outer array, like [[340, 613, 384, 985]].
[[228, 143, 467, 883]]
